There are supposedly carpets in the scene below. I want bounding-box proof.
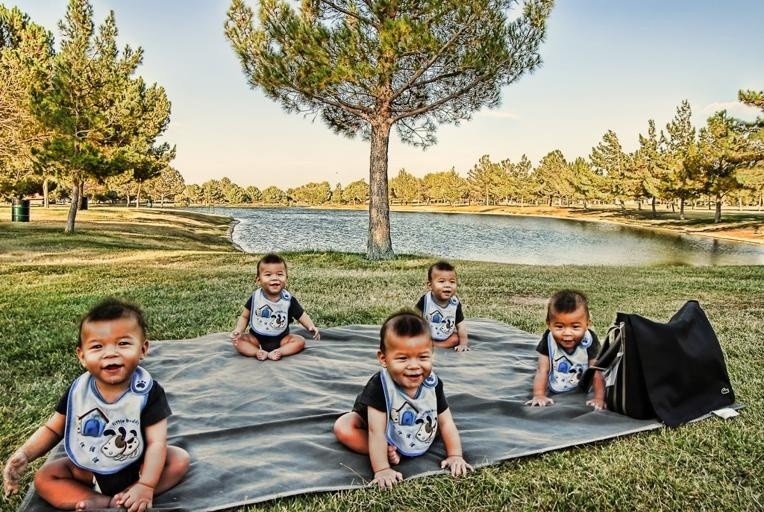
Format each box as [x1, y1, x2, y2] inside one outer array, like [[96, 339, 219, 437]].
[[9, 318, 747, 512]]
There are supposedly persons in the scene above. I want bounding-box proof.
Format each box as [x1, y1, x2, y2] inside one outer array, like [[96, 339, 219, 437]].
[[3, 299, 190, 512], [333, 307, 475, 490], [415, 262, 470, 351], [230, 254, 321, 361], [524, 290, 607, 411]]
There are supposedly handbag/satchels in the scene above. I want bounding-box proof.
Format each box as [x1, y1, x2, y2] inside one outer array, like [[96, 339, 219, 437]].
[[578, 300, 735, 428]]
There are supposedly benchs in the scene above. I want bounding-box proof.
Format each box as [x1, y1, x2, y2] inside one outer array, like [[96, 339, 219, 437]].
[[23, 198, 44, 207]]
